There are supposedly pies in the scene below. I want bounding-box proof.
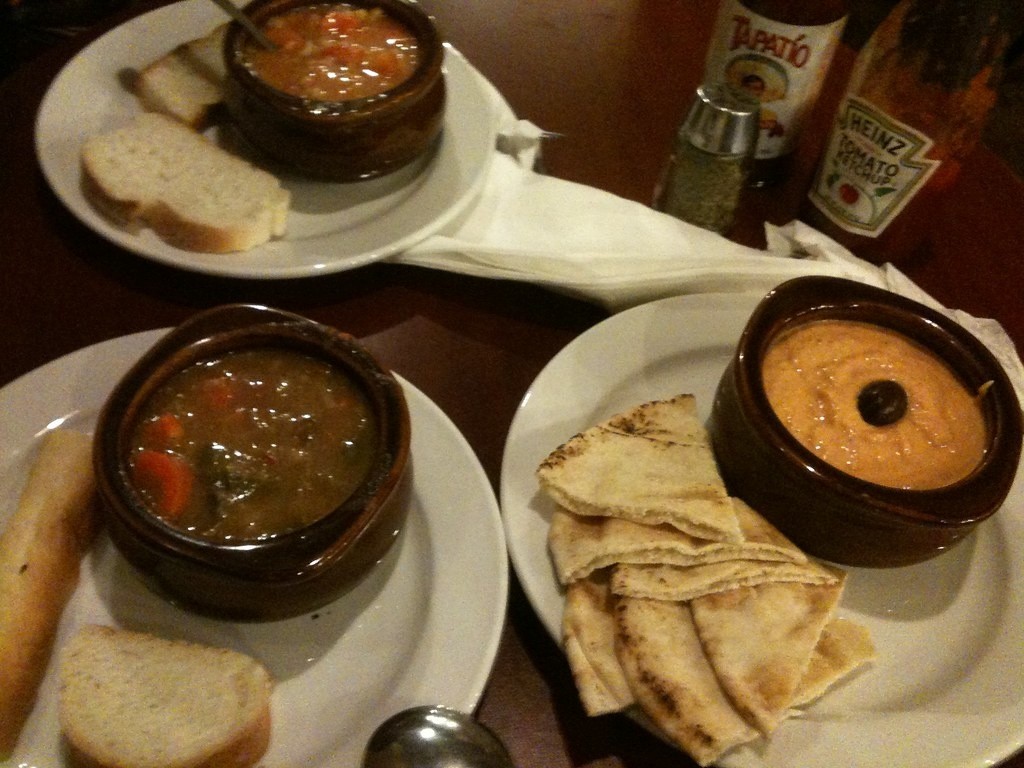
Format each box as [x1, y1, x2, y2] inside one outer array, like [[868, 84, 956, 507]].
[[535, 388, 879, 768]]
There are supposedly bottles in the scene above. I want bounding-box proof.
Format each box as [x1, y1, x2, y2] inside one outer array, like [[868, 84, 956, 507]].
[[652, 83, 760, 235], [687, 0, 859, 190], [799, 0, 1024, 269]]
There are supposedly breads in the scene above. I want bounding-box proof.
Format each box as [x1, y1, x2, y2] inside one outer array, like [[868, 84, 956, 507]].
[[57, 623, 273, 768], [0, 428, 106, 761], [133, 21, 228, 134], [78, 112, 292, 253]]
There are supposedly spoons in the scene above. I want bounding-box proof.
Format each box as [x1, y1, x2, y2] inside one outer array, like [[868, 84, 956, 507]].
[[359, 705, 512, 768]]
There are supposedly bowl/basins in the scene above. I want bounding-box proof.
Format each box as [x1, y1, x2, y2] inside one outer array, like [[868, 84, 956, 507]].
[[223, 1, 445, 184], [704, 275, 1023, 567], [87, 301, 414, 621]]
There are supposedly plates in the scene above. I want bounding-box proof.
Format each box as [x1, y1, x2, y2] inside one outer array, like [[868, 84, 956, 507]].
[[31, 0, 499, 281], [496, 288, 1024, 768], [1, 328, 510, 768]]
